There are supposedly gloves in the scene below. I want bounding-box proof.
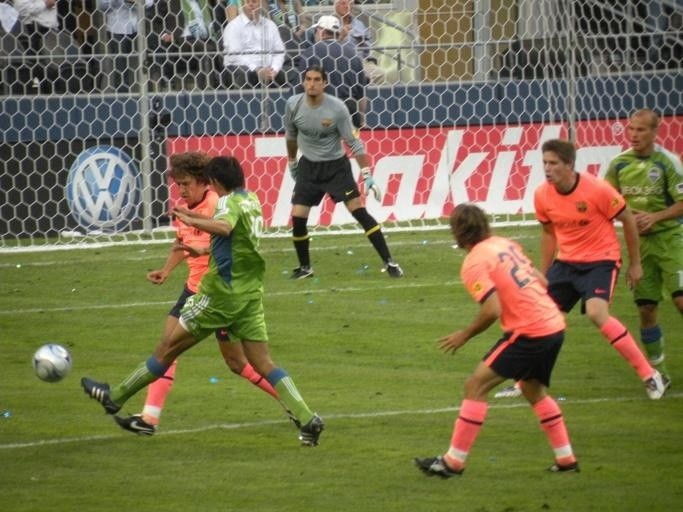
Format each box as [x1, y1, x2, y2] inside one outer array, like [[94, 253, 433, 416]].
[[361, 167, 381, 201], [286, 157, 297, 180]]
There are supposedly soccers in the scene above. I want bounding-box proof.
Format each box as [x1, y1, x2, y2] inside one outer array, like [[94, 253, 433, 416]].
[[33, 345, 72, 382]]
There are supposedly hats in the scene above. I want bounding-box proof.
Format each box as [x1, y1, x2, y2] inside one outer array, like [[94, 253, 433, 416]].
[[307, 15, 341, 33]]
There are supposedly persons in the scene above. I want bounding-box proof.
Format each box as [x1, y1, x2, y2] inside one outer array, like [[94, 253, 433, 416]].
[[113, 151, 304, 438], [314, 0, 372, 59], [79, 156, 325, 448], [604, 107, 683, 389], [411, 202, 579, 479], [494, 140, 671, 401], [301, 15, 366, 129], [284, 66, 404, 281]]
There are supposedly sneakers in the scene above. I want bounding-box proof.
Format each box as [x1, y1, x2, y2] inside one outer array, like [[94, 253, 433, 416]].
[[381, 263, 403, 279], [81, 377, 121, 415], [494, 387, 523, 399], [113, 415, 156, 437], [661, 371, 672, 389], [413, 456, 464, 479], [646, 370, 666, 402], [544, 462, 580, 474], [289, 266, 314, 281], [301, 414, 323, 447]]
